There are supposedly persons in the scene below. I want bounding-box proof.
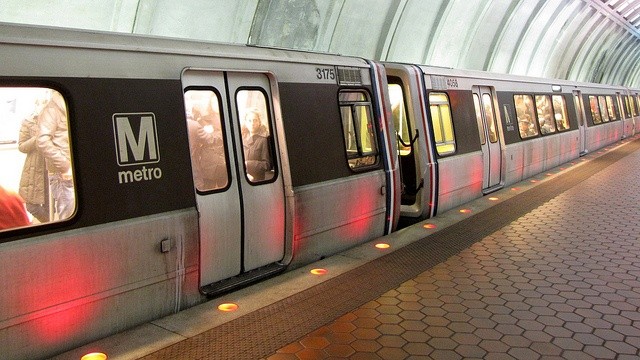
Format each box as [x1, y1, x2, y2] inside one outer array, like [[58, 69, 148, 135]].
[[36, 90, 76, 221], [18, 90, 60, 223], [514, 94, 567, 139], [198, 94, 227, 190], [0, 187, 32, 230], [240, 111, 274, 182], [185, 93, 228, 191]]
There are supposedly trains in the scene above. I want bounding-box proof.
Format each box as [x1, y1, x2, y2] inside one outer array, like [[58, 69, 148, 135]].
[[1, 22, 639, 360]]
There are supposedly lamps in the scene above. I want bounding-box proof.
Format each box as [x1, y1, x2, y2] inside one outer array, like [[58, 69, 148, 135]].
[[488, 196, 499, 201], [311, 268, 328, 275], [604, 148, 609, 151], [581, 158, 586, 162], [218, 303, 239, 312], [80, 352, 107, 360], [597, 151, 603, 155], [615, 143, 620, 146], [511, 187, 521, 191], [559, 167, 566, 171], [591, 155, 597, 159], [570, 162, 577, 165], [459, 209, 473, 214], [610, 145, 615, 148], [621, 141, 624, 144], [546, 173, 553, 176], [375, 243, 390, 249], [531, 180, 538, 183], [423, 224, 437, 229]]
